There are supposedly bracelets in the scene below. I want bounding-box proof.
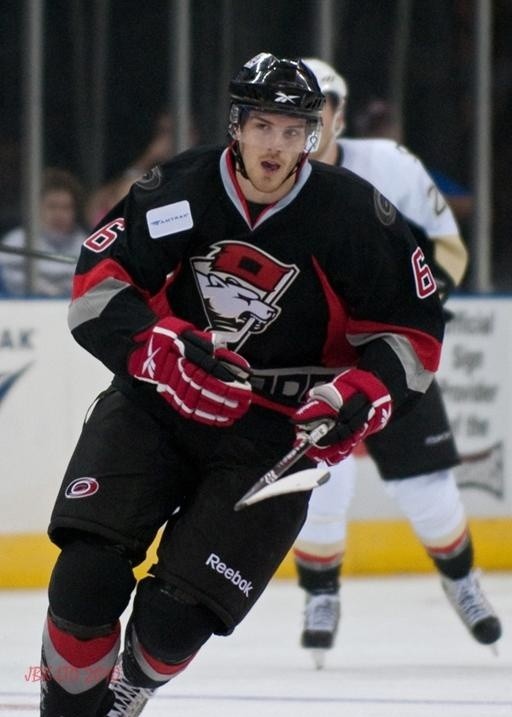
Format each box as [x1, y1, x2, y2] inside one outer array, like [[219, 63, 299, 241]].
[[123, 162, 148, 181]]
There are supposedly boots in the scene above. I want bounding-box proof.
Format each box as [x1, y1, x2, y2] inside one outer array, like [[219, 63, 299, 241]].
[[106, 654, 157, 716], [301, 591, 341, 647], [422, 570, 503, 645]]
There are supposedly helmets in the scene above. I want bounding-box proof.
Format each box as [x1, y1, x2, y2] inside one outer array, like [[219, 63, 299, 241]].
[[223, 51, 349, 153]]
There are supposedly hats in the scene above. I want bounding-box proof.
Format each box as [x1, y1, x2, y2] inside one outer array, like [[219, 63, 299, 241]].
[[40, 163, 85, 198]]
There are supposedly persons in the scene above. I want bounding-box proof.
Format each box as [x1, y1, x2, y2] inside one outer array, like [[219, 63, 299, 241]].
[[1, 172, 92, 298], [40, 50, 448, 715], [346, 95, 471, 214], [83, 102, 199, 239], [293, 54, 503, 651]]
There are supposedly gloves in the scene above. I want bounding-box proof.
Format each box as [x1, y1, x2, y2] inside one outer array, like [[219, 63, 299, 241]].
[[125, 317, 252, 429], [289, 366, 394, 470]]
